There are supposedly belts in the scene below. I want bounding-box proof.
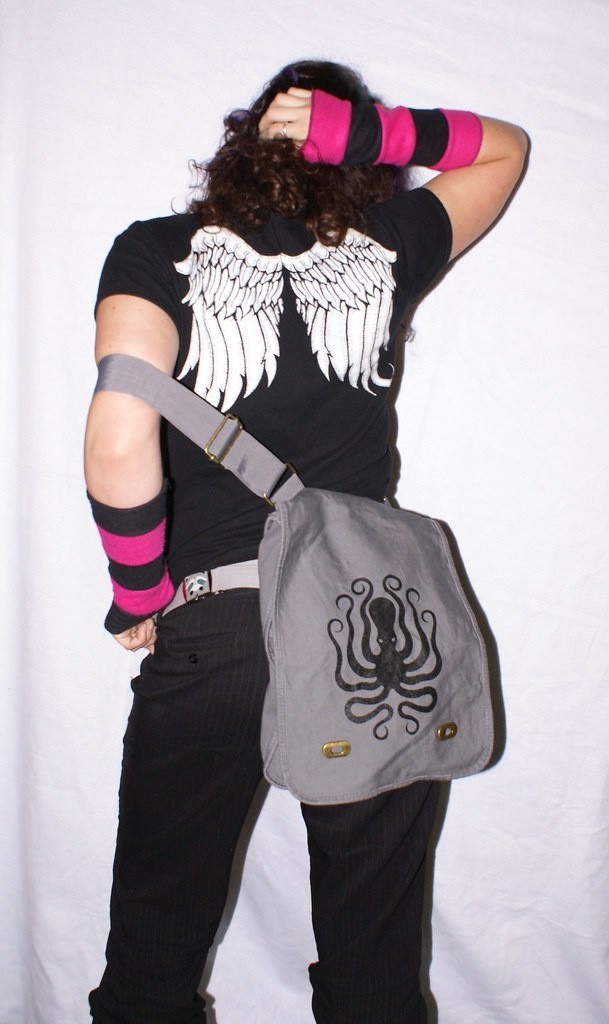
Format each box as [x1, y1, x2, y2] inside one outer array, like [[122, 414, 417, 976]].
[[161, 559, 260, 617]]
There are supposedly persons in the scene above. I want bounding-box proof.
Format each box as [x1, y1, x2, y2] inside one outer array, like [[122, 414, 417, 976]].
[[84, 59, 529, 1023]]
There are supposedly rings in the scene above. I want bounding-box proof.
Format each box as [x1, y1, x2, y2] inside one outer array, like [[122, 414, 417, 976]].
[[283, 122, 288, 138]]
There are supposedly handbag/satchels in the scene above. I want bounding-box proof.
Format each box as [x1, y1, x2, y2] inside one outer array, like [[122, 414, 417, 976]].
[[258, 488, 495, 806]]
